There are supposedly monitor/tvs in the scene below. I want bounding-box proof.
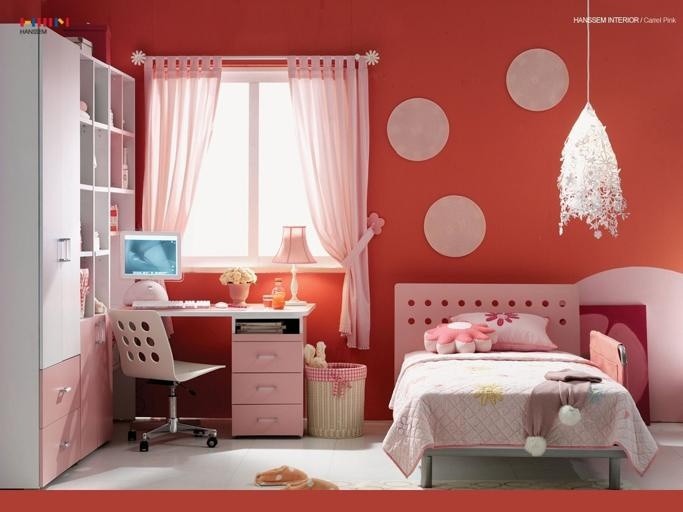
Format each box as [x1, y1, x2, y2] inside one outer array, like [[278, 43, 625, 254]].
[[119, 230, 184, 282]]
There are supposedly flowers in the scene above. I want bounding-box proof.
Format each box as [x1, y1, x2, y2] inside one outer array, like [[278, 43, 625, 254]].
[[219, 267, 257, 286]]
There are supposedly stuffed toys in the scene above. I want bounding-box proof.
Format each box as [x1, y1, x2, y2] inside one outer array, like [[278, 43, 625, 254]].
[[303, 340, 328, 368]]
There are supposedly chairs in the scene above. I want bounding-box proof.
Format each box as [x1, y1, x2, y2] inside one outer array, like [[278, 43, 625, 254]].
[[110, 309, 226, 451]]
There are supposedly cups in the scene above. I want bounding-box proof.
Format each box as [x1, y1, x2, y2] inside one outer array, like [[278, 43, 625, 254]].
[[261, 295, 273, 307]]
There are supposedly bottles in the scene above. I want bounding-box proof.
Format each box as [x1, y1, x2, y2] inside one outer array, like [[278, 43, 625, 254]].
[[273, 278, 285, 310]]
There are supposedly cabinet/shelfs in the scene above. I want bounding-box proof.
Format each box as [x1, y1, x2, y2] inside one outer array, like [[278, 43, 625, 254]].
[[0, 22, 135, 489]]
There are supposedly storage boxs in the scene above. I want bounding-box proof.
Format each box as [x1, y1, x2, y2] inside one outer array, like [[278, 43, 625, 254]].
[[52, 25, 111, 65], [63, 36, 93, 57]]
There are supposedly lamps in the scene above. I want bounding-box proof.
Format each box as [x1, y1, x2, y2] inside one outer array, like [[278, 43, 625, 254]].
[[272, 226, 317, 306], [557, 0, 632, 240]]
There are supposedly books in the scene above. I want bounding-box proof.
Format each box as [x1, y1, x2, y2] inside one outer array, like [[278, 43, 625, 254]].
[[234, 321, 286, 334]]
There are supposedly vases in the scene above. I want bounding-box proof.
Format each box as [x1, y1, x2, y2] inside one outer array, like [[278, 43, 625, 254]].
[[227, 283, 251, 307]]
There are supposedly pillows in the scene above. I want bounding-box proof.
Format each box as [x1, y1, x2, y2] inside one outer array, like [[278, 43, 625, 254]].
[[450, 312, 558, 352], [424, 321, 497, 355]]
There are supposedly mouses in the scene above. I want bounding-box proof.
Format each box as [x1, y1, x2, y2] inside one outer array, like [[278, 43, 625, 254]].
[[214, 302, 228, 309]]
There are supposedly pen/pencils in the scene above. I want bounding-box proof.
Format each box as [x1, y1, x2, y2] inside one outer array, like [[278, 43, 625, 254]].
[[228, 304, 247, 308]]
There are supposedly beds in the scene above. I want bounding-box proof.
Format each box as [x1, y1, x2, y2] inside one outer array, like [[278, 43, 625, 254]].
[[393, 283, 628, 492]]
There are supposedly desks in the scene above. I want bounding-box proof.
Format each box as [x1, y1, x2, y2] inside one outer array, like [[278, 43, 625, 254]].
[[111, 302, 317, 438]]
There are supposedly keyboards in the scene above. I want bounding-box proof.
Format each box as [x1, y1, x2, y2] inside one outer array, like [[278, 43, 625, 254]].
[[132, 299, 211, 310]]
